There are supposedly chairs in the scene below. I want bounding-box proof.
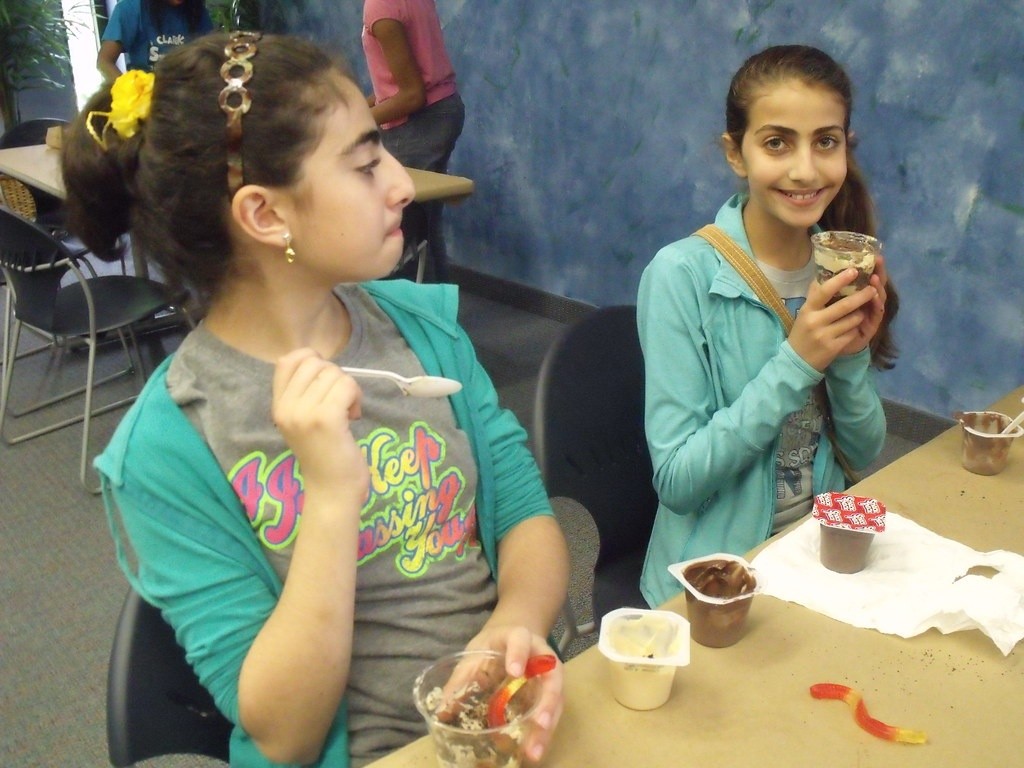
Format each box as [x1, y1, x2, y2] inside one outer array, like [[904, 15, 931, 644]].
[[536, 307, 660, 658], [104, 568, 240, 768], [1, 118, 191, 493]]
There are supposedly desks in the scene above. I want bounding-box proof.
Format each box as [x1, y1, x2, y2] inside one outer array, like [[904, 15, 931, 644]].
[[0, 135, 476, 354]]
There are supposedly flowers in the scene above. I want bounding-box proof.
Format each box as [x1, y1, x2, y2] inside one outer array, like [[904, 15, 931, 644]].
[[84, 68, 156, 148]]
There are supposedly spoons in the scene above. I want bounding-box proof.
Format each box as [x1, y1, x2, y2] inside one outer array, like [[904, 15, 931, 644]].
[[337, 364, 464, 401]]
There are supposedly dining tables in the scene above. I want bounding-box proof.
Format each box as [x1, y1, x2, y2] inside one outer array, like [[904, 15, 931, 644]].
[[358, 383, 1024, 768]]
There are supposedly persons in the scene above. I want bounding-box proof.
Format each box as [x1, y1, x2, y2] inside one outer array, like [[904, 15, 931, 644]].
[[95, 0, 215, 80], [361, 0, 466, 286], [63, 32, 571, 768], [635, 44, 897, 611]]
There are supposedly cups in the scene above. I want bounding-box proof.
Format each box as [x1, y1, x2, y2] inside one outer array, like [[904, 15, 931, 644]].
[[953, 409, 1024, 476], [596, 605, 692, 711], [413, 649, 546, 767], [811, 230, 885, 310], [667, 551, 769, 650], [813, 491, 887, 576]]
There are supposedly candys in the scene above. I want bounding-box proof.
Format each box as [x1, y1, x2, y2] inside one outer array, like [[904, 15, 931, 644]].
[[809, 683, 928, 743], [487, 654, 556, 755]]
